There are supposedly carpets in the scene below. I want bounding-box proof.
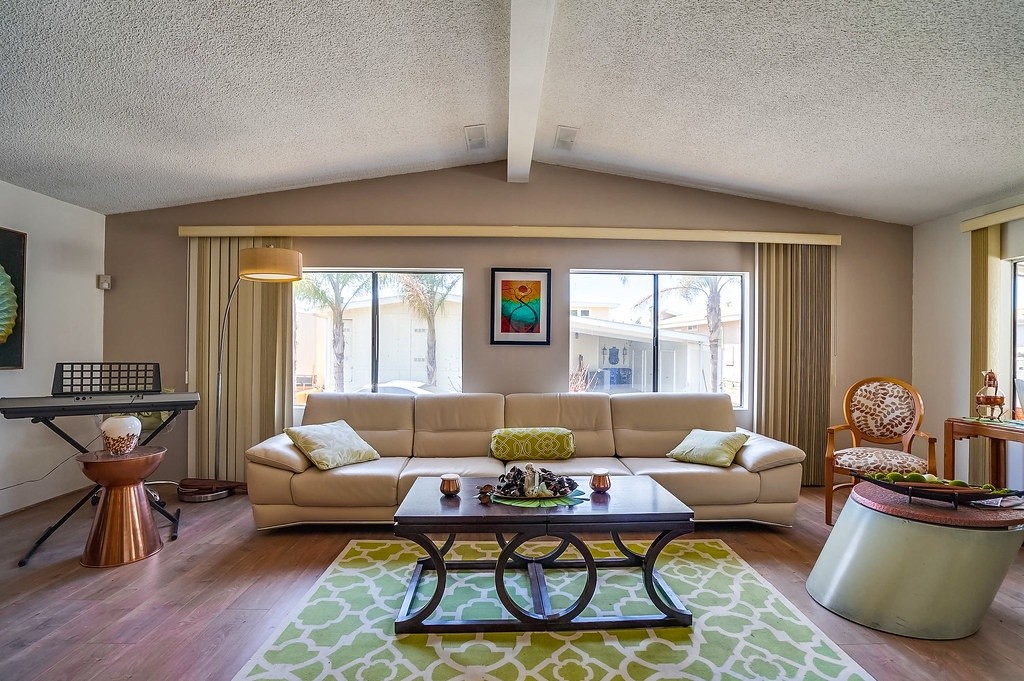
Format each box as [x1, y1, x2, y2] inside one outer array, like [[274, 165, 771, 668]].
[[230, 531, 881, 681]]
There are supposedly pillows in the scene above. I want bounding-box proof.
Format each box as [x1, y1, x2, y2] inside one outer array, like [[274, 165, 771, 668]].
[[281, 418, 381, 470], [490, 428, 575, 460], [666, 427, 751, 467]]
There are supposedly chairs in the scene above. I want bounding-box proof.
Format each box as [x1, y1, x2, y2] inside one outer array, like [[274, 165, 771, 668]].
[[821, 374, 941, 526]]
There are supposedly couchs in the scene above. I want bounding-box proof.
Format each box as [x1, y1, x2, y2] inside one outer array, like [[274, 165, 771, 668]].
[[244, 391, 806, 538]]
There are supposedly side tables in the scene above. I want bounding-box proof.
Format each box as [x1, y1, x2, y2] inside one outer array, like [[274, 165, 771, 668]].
[[75, 442, 170, 573]]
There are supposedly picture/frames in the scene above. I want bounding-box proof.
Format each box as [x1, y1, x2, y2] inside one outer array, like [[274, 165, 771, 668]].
[[0, 223, 27, 372], [488, 266, 555, 346]]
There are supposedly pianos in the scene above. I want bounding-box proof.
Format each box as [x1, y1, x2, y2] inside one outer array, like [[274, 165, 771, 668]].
[[0, 361, 201, 567]]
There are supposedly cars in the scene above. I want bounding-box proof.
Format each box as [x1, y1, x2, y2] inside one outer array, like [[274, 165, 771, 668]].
[[351, 380, 446, 395]]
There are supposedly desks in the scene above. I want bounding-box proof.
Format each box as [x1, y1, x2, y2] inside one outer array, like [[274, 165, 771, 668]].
[[943, 410, 1024, 488], [802, 481, 1024, 642]]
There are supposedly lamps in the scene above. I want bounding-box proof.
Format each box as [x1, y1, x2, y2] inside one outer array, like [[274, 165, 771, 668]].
[[100, 413, 143, 454], [175, 245, 306, 503]]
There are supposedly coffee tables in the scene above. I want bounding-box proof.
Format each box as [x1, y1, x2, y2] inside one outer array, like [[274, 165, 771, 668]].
[[390, 473, 700, 635]]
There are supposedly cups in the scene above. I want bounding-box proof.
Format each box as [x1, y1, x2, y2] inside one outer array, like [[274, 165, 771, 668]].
[[590, 469, 611, 493], [441, 474, 461, 497]]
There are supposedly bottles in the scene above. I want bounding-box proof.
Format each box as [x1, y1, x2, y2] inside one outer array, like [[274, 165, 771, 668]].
[[101, 416, 142, 457]]
